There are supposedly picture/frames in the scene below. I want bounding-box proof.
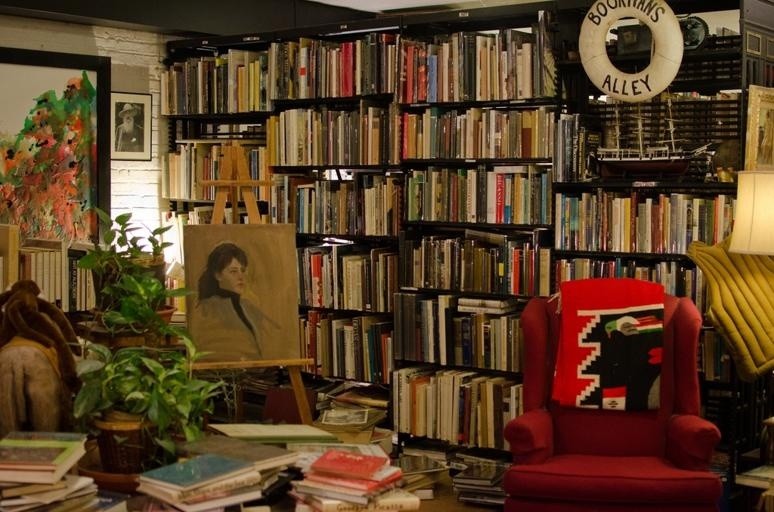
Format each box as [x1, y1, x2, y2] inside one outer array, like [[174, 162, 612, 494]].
[[2, 46, 112, 252], [109, 90, 153, 163], [745, 84, 774, 172]]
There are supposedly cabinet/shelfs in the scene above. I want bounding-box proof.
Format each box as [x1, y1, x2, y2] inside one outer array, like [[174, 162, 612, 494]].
[[159, 1, 745, 461]]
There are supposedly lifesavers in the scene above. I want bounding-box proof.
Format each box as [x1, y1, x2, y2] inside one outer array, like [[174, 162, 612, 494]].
[[579, 0, 684, 104]]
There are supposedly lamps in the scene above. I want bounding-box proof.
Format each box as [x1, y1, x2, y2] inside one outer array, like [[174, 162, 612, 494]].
[[728, 170, 774, 256]]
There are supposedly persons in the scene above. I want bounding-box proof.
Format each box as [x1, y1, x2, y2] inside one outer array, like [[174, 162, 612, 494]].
[[113, 104, 142, 153]]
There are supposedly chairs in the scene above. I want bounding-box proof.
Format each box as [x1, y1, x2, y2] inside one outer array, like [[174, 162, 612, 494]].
[[499, 275, 724, 510]]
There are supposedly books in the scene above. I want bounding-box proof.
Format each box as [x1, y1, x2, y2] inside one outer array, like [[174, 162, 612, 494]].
[[0, 238, 100, 316], [159, 27, 772, 452], [1, 420, 513, 512]]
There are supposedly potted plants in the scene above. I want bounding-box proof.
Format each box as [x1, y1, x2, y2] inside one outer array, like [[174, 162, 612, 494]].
[[69, 205, 241, 473]]
